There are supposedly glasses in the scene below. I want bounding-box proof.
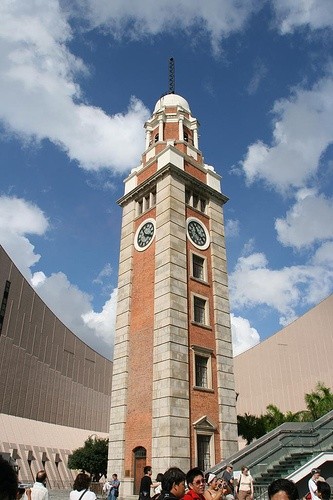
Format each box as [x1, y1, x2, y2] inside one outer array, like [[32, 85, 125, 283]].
[[191, 479, 205, 485]]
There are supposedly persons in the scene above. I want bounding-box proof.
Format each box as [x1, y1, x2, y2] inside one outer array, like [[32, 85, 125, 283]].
[[138, 466, 159, 500], [222, 463, 234, 496], [20, 471, 48, 500], [204, 472, 234, 500], [69, 473, 97, 500], [236, 466, 254, 500], [152, 467, 187, 500], [107, 474, 120, 500], [308, 468, 331, 500], [180, 467, 207, 500], [268, 478, 299, 500], [154, 473, 163, 494], [0, 455, 31, 500]]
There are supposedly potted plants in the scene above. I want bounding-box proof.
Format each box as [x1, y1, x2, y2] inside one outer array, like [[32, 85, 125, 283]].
[[67, 433, 110, 496]]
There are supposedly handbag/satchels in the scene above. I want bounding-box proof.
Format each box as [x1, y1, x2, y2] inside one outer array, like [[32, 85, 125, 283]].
[[114, 490, 119, 497]]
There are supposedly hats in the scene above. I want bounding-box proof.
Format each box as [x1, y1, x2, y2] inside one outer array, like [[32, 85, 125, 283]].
[[226, 463, 235, 468]]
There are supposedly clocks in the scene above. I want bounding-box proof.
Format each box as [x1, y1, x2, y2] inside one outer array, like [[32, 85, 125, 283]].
[[136, 222, 155, 247], [187, 221, 206, 246]]
